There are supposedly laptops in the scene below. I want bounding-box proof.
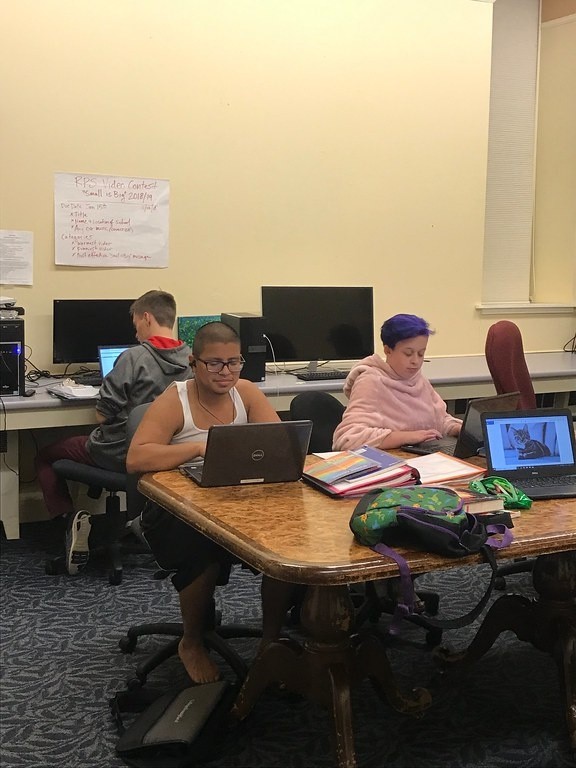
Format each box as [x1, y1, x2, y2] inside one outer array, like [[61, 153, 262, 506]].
[[97, 345, 136, 380], [401, 391, 522, 459], [479, 408, 576, 502], [179, 420, 313, 488]]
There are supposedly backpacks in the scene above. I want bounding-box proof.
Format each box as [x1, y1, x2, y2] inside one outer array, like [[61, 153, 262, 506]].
[[349, 485, 514, 635]]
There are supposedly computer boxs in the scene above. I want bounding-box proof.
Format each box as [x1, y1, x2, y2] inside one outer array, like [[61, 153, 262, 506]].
[[221, 311, 266, 382], [0, 316, 25, 396]]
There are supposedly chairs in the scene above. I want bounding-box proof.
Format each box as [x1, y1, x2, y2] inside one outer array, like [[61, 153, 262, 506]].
[[53, 390, 396, 702], [134, 448, 576, 768], [487, 321, 537, 409]]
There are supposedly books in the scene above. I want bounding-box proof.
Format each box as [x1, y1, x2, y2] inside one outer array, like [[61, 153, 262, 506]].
[[439, 485, 521, 519], [302, 444, 420, 499], [406, 451, 489, 486]]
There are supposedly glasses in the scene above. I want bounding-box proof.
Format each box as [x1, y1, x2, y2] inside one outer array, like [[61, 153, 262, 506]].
[[192, 353, 246, 373]]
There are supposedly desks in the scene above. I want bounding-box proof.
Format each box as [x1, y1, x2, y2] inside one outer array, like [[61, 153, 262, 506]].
[[0, 351, 575, 544]]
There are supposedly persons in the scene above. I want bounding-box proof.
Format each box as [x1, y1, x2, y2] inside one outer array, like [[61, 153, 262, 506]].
[[331, 314, 463, 613], [33, 290, 194, 543], [126, 320, 284, 684]]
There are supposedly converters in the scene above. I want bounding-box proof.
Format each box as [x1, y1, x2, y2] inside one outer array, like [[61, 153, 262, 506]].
[[0, 431, 8, 453]]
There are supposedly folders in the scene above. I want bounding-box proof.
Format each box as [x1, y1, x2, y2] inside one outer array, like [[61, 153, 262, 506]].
[[302, 463, 421, 498]]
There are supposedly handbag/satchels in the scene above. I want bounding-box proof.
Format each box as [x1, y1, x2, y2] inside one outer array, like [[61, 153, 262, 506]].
[[110, 681, 249, 768]]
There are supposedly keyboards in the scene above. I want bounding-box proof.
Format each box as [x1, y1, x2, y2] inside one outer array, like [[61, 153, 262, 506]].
[[73, 377, 103, 386], [297, 371, 349, 381]]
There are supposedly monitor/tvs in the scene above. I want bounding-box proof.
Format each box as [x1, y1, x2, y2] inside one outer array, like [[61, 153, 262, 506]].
[[53, 298, 141, 364], [261, 285, 374, 373]]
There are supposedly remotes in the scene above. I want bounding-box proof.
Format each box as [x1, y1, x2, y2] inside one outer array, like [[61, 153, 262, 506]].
[[24, 390, 36, 396]]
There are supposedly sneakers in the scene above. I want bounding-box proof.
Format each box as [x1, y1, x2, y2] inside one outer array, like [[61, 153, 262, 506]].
[[64, 510, 93, 575], [118, 527, 137, 547]]
